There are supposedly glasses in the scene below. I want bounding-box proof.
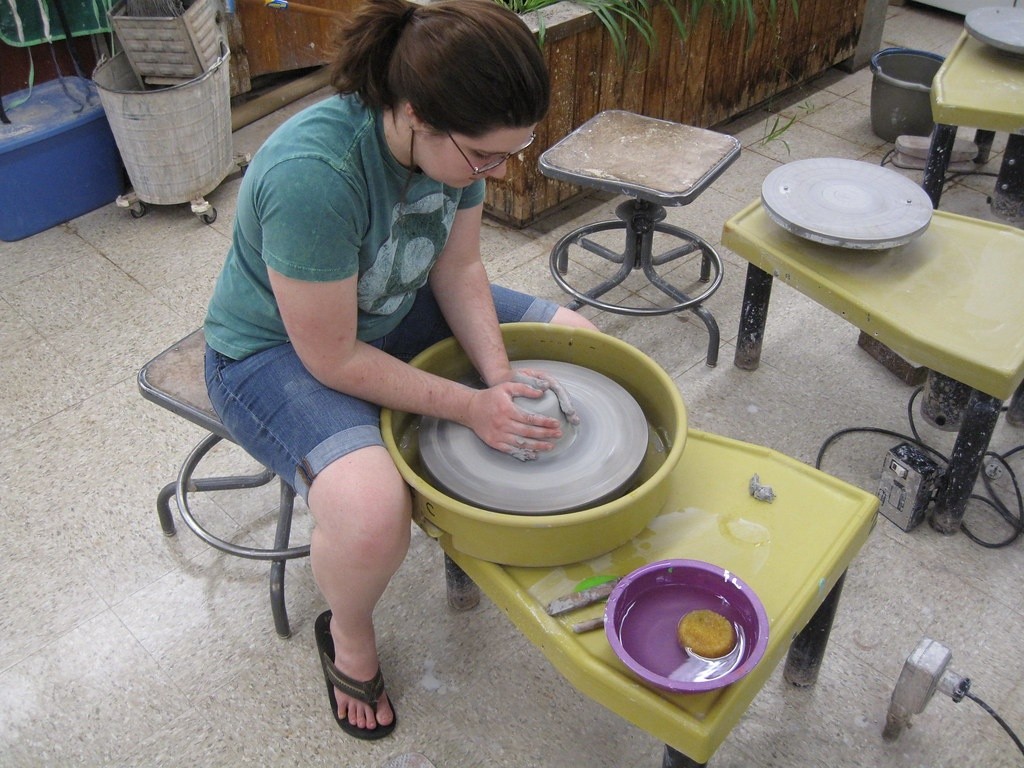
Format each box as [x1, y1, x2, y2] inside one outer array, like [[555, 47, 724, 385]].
[[445, 128, 538, 177]]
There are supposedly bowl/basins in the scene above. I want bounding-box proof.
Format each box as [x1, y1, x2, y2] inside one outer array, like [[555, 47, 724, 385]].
[[604, 557, 767, 695]]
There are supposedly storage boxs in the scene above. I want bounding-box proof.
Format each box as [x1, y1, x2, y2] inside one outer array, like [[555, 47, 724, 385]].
[[0, 75, 127, 242]]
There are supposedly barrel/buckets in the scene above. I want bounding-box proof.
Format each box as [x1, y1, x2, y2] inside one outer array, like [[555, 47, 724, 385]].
[[870, 48, 948, 144]]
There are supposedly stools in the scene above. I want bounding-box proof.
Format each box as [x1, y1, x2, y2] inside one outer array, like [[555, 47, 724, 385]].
[[536, 109, 745, 369], [137, 323, 311, 640]]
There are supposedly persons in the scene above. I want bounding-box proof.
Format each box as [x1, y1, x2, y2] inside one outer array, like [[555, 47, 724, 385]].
[[204, 1, 600, 740]]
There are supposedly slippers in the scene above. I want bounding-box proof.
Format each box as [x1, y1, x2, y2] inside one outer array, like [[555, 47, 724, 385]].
[[313, 607, 397, 741]]
